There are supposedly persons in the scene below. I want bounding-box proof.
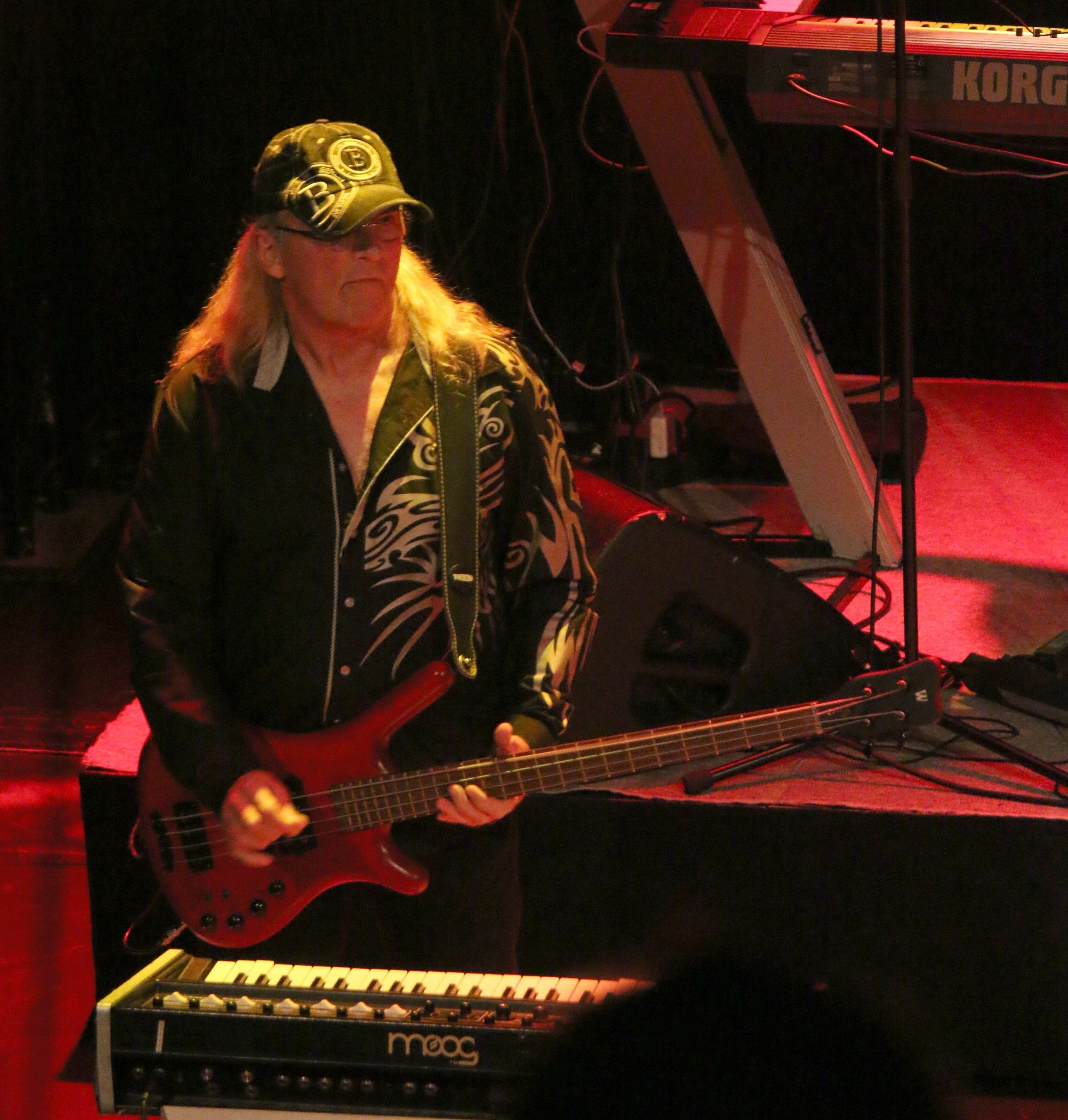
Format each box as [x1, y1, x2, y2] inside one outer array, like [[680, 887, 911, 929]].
[[114, 116, 597, 979]]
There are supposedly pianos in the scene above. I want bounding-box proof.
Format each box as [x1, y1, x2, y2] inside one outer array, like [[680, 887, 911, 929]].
[[90, 949, 663, 1111]]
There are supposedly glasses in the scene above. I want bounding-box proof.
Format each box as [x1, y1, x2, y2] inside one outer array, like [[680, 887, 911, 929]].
[[275, 207, 408, 249]]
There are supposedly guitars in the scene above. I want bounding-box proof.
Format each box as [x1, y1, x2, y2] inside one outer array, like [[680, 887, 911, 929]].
[[137, 654, 950, 949]]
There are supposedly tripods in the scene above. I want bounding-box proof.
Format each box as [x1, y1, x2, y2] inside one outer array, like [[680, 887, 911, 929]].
[[680, 0, 1068, 796]]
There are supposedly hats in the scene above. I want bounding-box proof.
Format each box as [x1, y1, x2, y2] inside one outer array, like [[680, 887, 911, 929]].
[[254, 119, 433, 238]]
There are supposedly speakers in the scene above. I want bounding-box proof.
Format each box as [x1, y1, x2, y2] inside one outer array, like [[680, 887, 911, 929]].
[[565, 466, 887, 740]]
